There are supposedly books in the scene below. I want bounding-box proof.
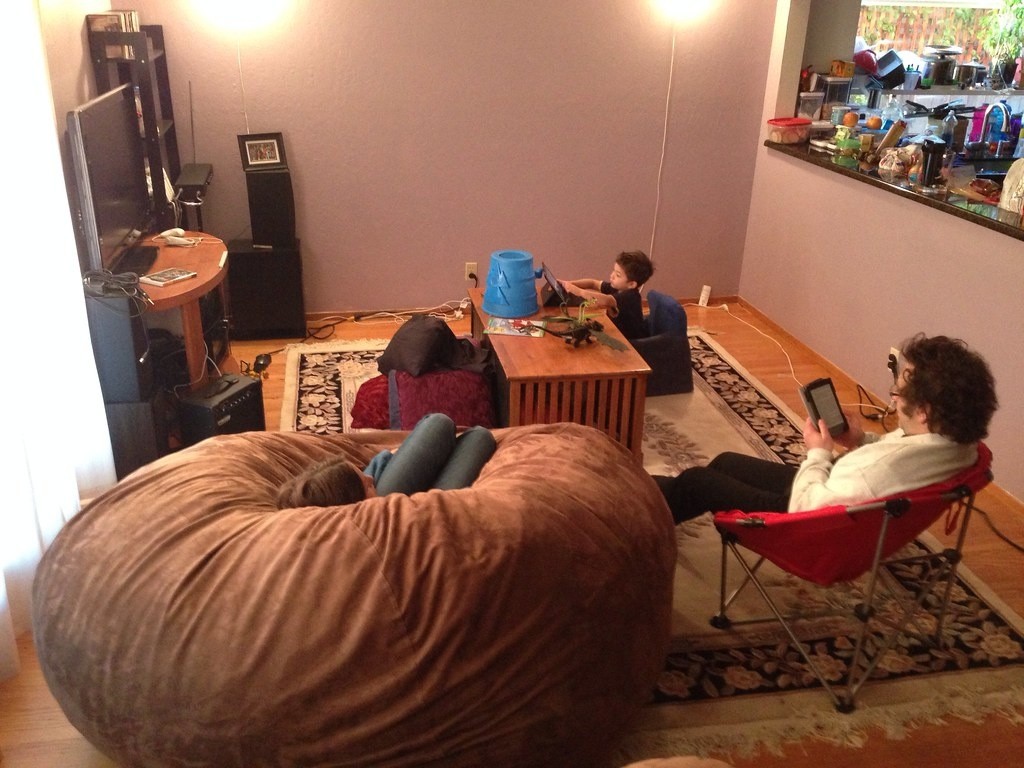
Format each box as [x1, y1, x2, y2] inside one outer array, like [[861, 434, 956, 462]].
[[86, 8, 142, 60], [133, 85, 160, 139], [138, 267, 197, 287], [144, 166, 175, 204], [483, 317, 547, 338]]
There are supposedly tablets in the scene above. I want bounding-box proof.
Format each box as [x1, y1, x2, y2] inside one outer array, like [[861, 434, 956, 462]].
[[542, 261, 566, 305], [804, 377, 851, 437]]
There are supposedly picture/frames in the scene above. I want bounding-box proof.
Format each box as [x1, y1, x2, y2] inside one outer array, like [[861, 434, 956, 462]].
[[237, 133, 287, 171]]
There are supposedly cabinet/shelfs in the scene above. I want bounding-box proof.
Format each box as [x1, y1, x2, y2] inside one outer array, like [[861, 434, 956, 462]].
[[92, 25, 189, 230], [846, 76, 1024, 190]]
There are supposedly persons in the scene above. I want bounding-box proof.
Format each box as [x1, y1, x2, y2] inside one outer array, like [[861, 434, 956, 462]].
[[555, 250, 654, 340], [276, 413, 497, 509], [648, 331, 999, 525]]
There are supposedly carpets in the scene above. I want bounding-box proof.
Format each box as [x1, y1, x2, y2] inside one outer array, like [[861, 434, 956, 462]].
[[281, 325, 1024, 768]]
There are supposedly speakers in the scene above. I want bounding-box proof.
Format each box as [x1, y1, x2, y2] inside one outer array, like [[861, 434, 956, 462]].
[[87, 288, 154, 403], [246, 168, 296, 248], [225, 238, 306, 341], [105, 386, 166, 486], [178, 372, 266, 449]]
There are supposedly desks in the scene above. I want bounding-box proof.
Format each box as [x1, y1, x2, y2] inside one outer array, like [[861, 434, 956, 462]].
[[466, 286, 652, 466], [133, 231, 231, 395]]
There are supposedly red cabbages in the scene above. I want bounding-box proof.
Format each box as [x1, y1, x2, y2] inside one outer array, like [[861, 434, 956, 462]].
[[853, 49, 878, 73]]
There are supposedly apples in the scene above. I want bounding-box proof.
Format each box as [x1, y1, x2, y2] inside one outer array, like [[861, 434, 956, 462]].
[[866, 116, 882, 129], [843, 112, 858, 127]]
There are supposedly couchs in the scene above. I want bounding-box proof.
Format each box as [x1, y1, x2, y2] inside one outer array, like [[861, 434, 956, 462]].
[[32, 423, 677, 768]]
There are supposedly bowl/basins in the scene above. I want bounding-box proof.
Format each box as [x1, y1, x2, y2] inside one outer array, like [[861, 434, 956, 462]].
[[768, 117, 812, 144]]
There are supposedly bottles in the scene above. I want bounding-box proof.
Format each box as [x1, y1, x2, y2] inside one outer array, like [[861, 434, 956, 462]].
[[882, 95, 902, 126], [969, 102, 993, 143], [986, 100, 1012, 143], [942, 110, 958, 152], [921, 137, 946, 187]]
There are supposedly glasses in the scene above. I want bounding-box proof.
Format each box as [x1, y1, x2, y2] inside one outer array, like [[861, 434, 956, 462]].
[[889, 385, 906, 400]]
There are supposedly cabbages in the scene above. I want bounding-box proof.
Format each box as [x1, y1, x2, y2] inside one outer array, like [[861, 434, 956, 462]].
[[834, 124, 856, 141]]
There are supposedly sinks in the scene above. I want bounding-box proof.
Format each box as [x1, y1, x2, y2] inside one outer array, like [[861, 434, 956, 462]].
[[959, 157, 1022, 184]]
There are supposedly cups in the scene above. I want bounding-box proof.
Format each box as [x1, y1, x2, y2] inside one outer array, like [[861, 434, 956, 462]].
[[831, 106, 852, 125], [903, 71, 922, 90]]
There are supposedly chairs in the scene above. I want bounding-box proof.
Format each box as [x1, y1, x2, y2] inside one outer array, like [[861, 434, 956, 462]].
[[628, 291, 694, 397], [708, 441, 994, 714]]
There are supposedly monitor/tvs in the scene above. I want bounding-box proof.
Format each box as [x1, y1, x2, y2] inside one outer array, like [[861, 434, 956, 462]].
[[61, 82, 160, 281]]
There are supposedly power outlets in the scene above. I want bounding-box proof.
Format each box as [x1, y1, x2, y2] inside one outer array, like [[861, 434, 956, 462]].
[[888, 346, 900, 374], [465, 262, 477, 281]]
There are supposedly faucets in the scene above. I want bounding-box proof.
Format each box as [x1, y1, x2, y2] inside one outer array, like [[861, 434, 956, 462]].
[[978, 101, 1012, 144]]
